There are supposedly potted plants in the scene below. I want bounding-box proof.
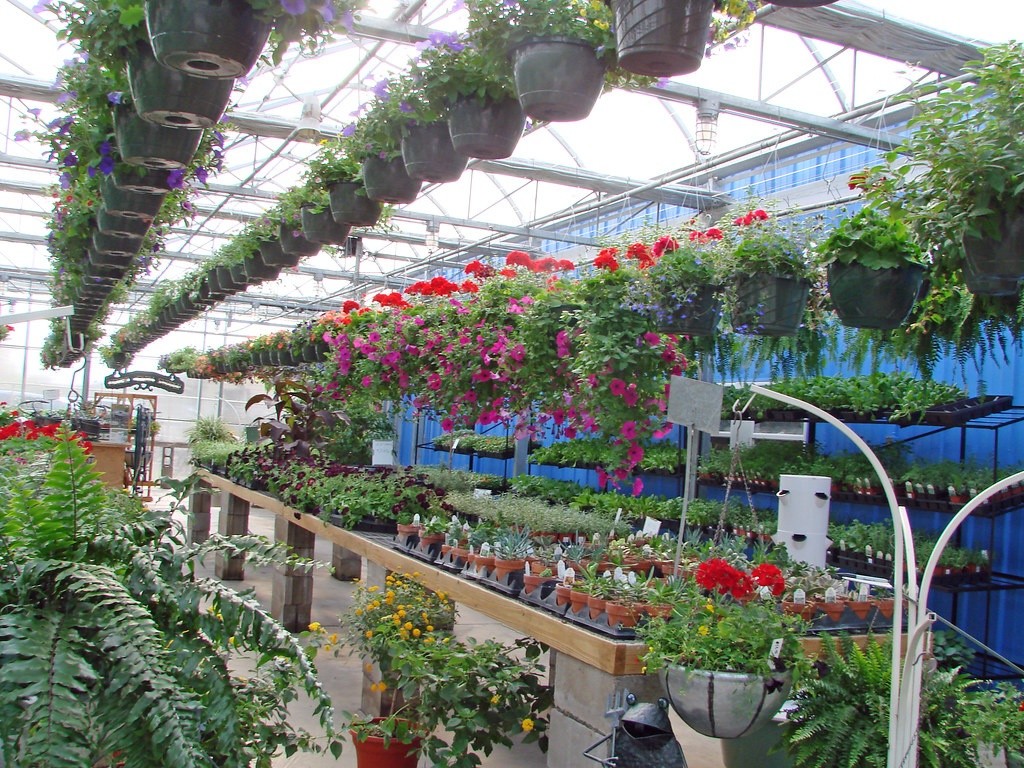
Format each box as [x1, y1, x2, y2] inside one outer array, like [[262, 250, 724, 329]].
[[960, 40, 1024, 297], [395, 512, 909, 629], [434, 375, 1011, 585]]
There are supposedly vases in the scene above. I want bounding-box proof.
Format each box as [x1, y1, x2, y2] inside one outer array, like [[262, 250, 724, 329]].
[[278, 224, 323, 257], [182, 293, 206, 312], [259, 242, 298, 268], [168, 306, 191, 321], [55, 305, 98, 368], [124, 43, 235, 130], [300, 202, 351, 245], [87, 263, 124, 281], [290, 348, 301, 363], [259, 351, 270, 366], [187, 369, 210, 379], [89, 248, 133, 269], [347, 718, 430, 768], [99, 179, 165, 220], [604, 0, 715, 78], [166, 368, 187, 374], [217, 362, 226, 374], [206, 270, 235, 295], [79, 285, 113, 295], [362, 154, 422, 204], [75, 298, 104, 307], [235, 360, 246, 372], [244, 251, 278, 282], [315, 341, 330, 362], [508, 36, 604, 121], [269, 350, 278, 365], [251, 352, 260, 368], [216, 266, 247, 291], [814, 204, 924, 329], [143, 0, 273, 81], [104, 353, 132, 369], [164, 311, 183, 324], [446, 91, 526, 160], [120, 322, 178, 355], [228, 362, 238, 372], [277, 351, 299, 367], [327, 182, 381, 228], [110, 166, 173, 196], [655, 284, 725, 335], [81, 276, 118, 287], [93, 231, 144, 257], [176, 303, 193, 314], [658, 660, 794, 738], [224, 364, 230, 373], [96, 209, 153, 239], [76, 288, 108, 300], [230, 265, 258, 285], [727, 272, 813, 337], [117, 108, 204, 171], [399, 120, 468, 183], [301, 345, 317, 362]]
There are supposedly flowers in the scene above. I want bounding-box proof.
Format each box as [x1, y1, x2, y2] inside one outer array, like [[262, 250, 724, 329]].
[[184, 0, 761, 291], [99, 278, 183, 361], [159, 318, 324, 386], [39, 317, 65, 371], [637, 560, 810, 682], [325, 198, 766, 497], [297, 565, 557, 768], [0, 324, 16, 343], [0, 0, 361, 307], [725, 195, 813, 267], [0, 401, 94, 465]]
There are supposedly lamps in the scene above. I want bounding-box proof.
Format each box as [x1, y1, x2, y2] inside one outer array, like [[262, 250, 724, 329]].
[[425, 221, 439, 252], [297, 94, 322, 138], [694, 100, 721, 154]]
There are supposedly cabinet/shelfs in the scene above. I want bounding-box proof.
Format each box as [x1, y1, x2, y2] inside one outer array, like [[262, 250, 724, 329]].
[[94, 392, 158, 502]]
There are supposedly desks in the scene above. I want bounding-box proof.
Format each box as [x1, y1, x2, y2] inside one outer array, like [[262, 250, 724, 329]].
[[186, 466, 935, 768]]
